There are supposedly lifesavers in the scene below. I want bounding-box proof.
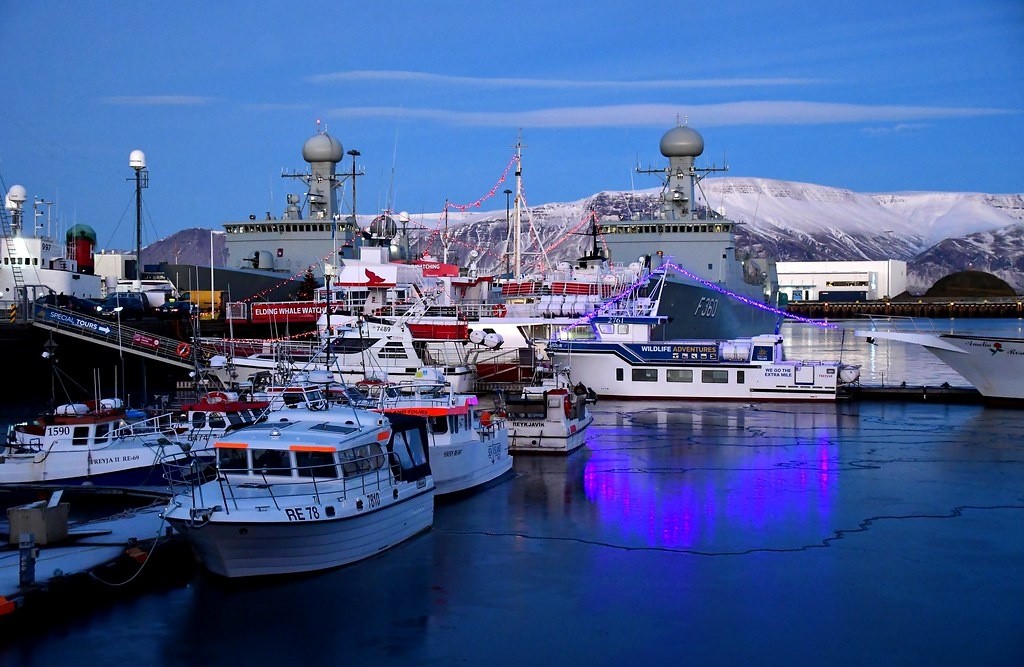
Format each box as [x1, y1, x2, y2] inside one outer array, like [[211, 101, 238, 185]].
[[493, 304, 507, 318], [177, 343, 190, 357], [564, 396, 570, 418], [205, 392, 228, 403]]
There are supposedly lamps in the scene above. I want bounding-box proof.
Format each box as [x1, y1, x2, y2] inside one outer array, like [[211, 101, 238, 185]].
[[270, 427, 279, 436]]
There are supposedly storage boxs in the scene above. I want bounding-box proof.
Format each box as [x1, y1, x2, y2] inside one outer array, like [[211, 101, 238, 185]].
[[7, 501, 70, 546]]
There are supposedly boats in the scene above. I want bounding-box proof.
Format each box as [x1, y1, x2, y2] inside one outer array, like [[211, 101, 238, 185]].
[[1, 116, 1024, 667]]
